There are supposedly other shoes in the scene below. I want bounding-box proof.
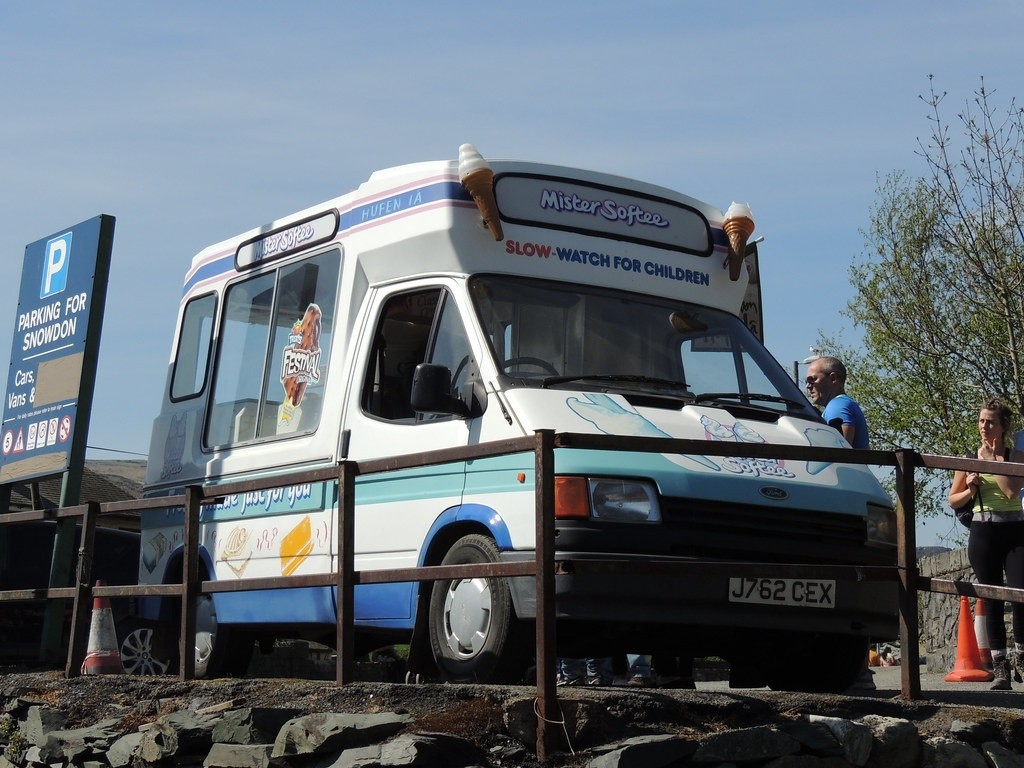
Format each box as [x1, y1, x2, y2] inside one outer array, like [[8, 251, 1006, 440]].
[[581, 672, 615, 685], [556, 667, 584, 686], [628, 675, 651, 688], [842, 665, 876, 690]]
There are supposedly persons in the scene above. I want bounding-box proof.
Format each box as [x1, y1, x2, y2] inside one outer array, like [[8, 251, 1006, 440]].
[[557, 655, 695, 689], [803, 355, 877, 690], [948, 398, 1024, 691]]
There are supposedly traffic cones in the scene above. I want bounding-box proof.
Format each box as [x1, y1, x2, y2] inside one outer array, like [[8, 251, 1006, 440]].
[[80, 580, 128, 674], [944, 593, 992, 683], [974, 596, 994, 671]]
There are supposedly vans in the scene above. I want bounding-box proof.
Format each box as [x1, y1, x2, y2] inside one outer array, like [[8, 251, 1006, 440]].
[[135, 144, 902, 682]]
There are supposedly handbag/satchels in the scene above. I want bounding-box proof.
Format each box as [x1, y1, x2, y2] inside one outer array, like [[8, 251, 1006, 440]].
[[954, 447, 978, 528]]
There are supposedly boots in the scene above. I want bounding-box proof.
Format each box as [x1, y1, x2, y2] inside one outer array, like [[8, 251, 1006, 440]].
[[990, 655, 1012, 690], [1014, 651, 1024, 683]]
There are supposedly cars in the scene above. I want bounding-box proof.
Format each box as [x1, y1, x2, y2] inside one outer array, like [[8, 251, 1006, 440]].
[[0, 483, 172, 674]]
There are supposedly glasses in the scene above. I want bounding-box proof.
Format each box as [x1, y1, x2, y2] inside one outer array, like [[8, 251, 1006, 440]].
[[806, 374, 830, 385]]
